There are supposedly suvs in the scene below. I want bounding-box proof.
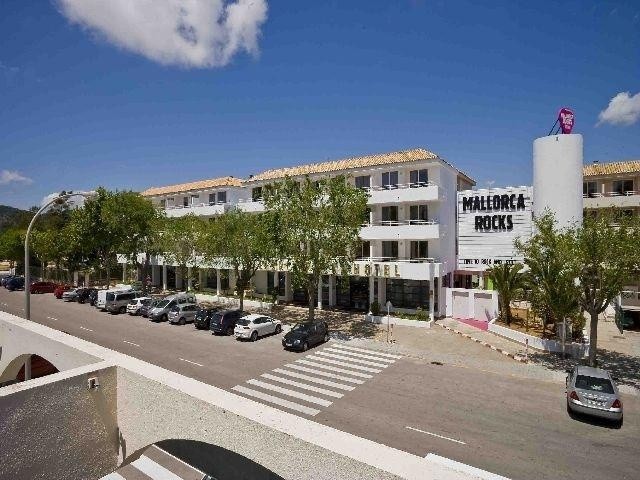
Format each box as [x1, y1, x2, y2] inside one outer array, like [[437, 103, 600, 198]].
[[0, 271, 283, 344], [283, 319, 328, 352]]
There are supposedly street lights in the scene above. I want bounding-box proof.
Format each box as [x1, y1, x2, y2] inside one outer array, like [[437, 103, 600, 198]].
[[23, 191, 98, 380]]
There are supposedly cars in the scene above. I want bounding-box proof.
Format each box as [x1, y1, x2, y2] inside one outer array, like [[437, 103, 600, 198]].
[[564, 364, 624, 421]]
